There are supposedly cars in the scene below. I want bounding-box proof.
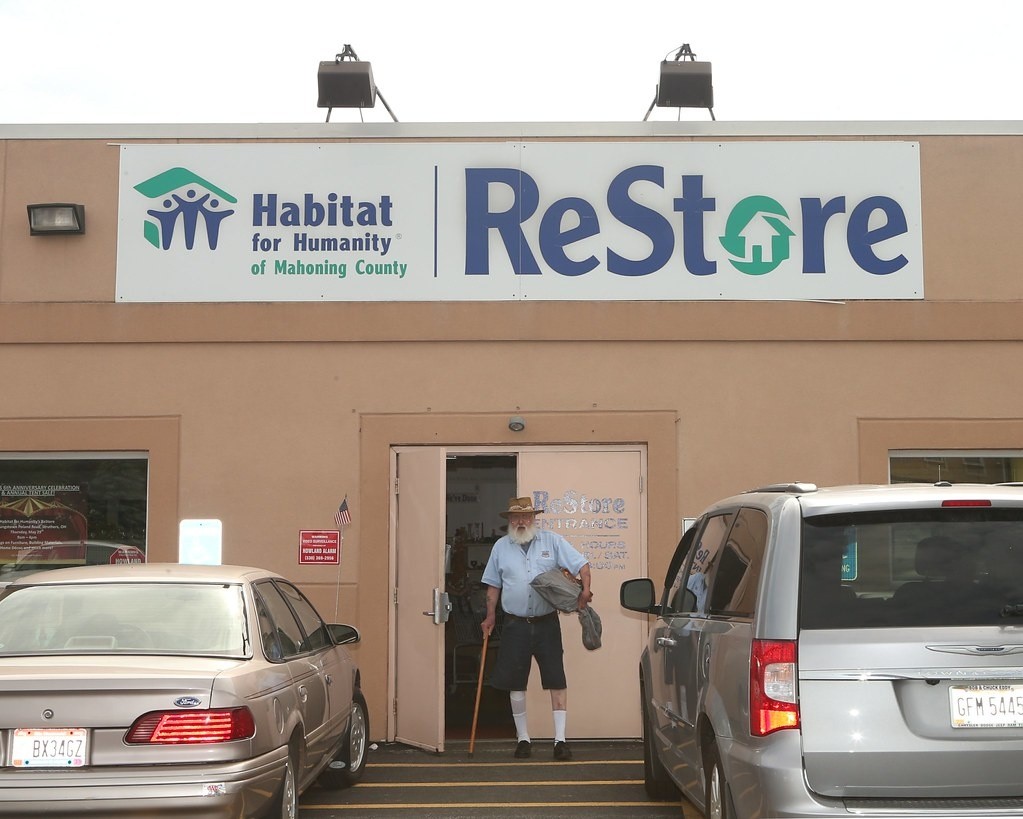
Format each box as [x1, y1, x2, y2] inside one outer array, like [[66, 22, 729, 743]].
[[0, 538, 145, 596], [0, 556, 371, 819]]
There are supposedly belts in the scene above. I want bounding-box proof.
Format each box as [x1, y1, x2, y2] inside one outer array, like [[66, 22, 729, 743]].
[[505, 610, 557, 624]]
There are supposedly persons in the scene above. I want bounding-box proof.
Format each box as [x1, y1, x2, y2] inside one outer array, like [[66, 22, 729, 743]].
[[480, 496, 591, 760]]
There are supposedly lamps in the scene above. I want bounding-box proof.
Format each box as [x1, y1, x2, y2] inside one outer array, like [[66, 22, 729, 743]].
[[27, 203, 84, 236], [509, 416, 525, 430], [318, 44, 398, 122], [642, 44, 715, 121]]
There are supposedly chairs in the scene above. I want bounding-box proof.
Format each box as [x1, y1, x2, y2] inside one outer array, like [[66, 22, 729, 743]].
[[893, 536, 992, 603]]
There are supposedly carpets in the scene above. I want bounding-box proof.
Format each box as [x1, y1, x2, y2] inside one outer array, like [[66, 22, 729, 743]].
[[445, 722, 515, 738]]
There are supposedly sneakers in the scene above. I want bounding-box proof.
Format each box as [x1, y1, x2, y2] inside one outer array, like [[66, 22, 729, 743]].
[[553, 740, 572, 759], [515, 740, 532, 757]]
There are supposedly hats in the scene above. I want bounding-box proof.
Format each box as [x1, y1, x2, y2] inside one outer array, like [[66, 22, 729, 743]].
[[499, 497, 544, 519]]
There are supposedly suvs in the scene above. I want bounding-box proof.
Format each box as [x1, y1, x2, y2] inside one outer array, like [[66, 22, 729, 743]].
[[617, 482, 1023, 819]]
